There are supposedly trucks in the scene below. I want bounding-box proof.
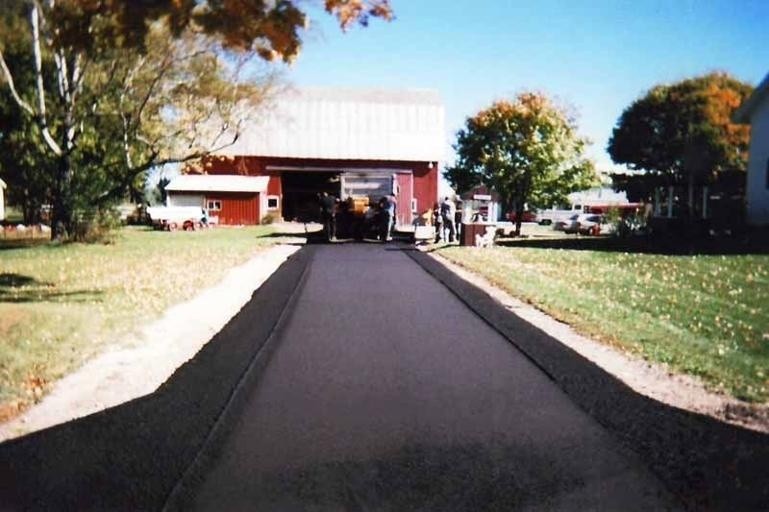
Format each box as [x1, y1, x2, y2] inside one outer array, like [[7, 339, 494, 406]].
[[530, 203, 585, 227], [328, 172, 400, 239], [144, 205, 219, 232]]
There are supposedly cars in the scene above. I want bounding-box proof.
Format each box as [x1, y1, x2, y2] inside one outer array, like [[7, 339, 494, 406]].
[[556, 214, 598, 233], [576, 214, 633, 237]]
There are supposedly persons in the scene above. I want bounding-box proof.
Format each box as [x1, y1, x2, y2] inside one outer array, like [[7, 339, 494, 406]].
[[434, 204, 442, 243], [454, 196, 488, 240], [441, 197, 457, 243], [318, 192, 396, 241], [413, 215, 422, 227], [423, 208, 435, 226]]
